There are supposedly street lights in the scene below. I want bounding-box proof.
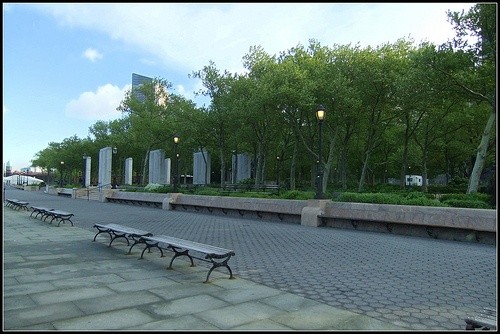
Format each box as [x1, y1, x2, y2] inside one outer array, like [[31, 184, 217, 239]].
[[111, 145, 118, 189], [408, 166, 411, 187], [27, 169, 29, 186], [316, 159, 319, 171], [276, 155, 280, 185], [82, 155, 86, 188], [315, 104, 326, 199], [60, 160, 64, 188], [173, 135, 179, 193]]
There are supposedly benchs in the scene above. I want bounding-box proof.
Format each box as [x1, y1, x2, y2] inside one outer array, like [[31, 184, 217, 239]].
[[139, 234, 236, 284], [6, 197, 75, 228], [92, 223, 153, 256], [217, 181, 280, 196], [464, 304, 496, 330]]
